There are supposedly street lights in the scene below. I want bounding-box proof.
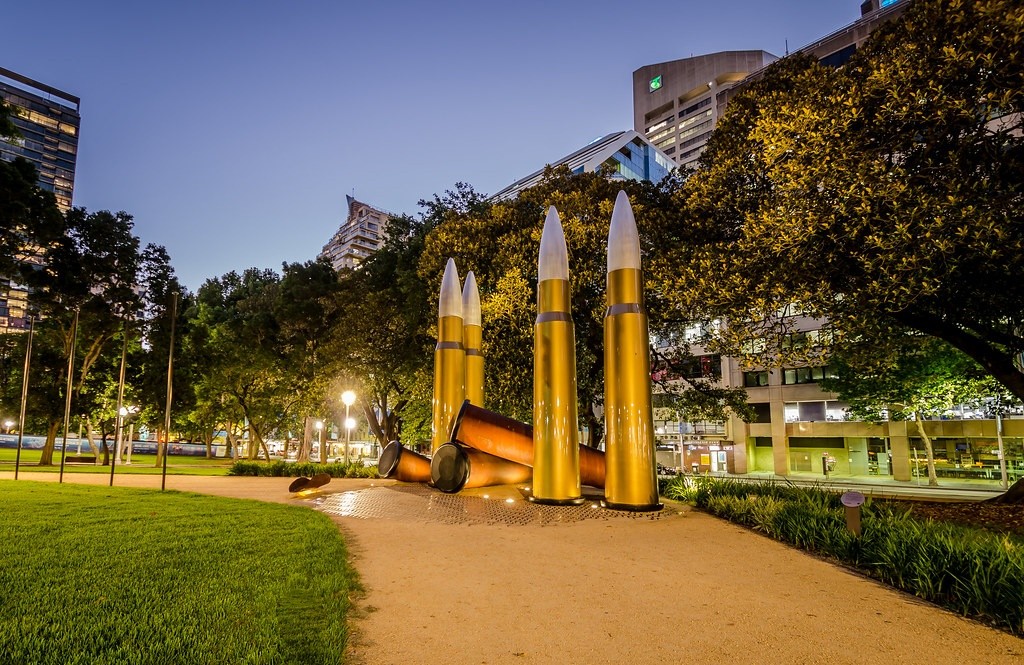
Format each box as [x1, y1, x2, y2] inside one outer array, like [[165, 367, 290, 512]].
[[115, 407, 128, 464], [317, 421, 321, 460], [339, 390, 356, 463]]
